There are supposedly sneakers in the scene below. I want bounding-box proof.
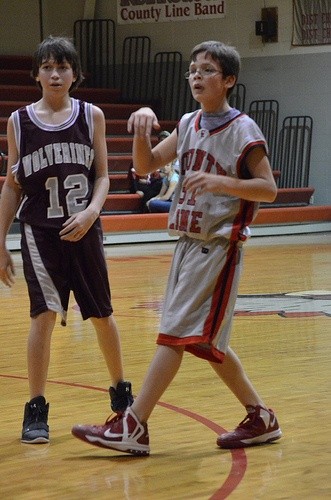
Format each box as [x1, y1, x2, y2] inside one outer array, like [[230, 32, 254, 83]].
[[72, 407, 150, 456], [21, 396, 50, 443], [109, 382, 136, 414], [217, 404, 282, 447]]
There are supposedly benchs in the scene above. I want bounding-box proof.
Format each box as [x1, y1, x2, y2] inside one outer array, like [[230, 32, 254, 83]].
[[0, 54, 331, 234]]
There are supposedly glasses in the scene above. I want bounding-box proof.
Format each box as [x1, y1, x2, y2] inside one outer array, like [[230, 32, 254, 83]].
[[185, 68, 228, 79]]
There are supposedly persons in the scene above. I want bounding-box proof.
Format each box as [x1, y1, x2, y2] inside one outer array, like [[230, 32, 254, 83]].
[[73, 41, 283, 455], [1, 34, 136, 443]]
